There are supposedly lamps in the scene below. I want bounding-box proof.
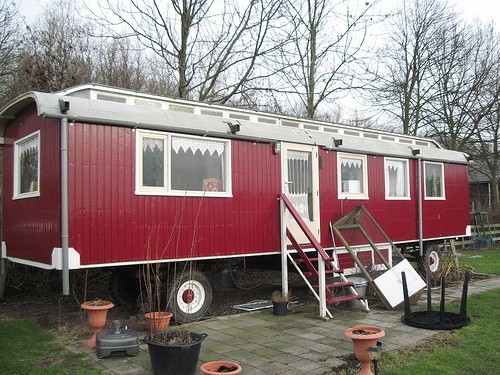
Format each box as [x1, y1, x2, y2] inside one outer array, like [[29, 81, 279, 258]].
[[366, 346, 381, 361], [274, 142, 281, 154]]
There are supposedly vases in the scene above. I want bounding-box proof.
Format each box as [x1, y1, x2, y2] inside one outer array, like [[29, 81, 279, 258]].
[[344, 326, 386, 375], [200, 361, 242, 375], [137, 330, 209, 375], [145, 312, 173, 331]]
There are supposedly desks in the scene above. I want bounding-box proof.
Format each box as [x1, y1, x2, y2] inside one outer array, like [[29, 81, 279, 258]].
[[401, 268, 471, 330]]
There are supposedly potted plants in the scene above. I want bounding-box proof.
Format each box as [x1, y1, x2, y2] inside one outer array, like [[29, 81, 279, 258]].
[[81, 298, 115, 347], [271, 290, 293, 316]]
